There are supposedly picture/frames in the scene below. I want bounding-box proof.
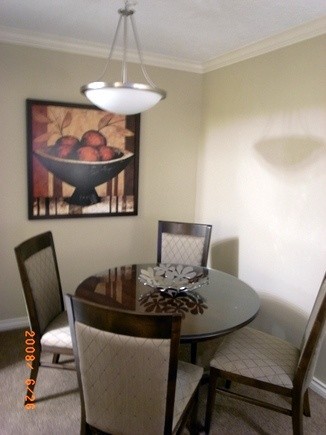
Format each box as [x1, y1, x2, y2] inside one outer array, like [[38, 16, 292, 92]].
[[26, 99, 141, 220]]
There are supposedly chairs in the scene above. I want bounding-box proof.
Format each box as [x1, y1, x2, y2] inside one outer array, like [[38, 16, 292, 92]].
[[14, 231, 77, 406], [64, 294, 205, 435], [204, 272, 326, 435], [157, 220, 212, 267]]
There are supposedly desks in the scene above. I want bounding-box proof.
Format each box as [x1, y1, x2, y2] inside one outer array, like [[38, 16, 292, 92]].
[[76, 264, 262, 364]]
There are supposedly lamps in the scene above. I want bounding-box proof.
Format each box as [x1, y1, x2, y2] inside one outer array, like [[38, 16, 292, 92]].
[[80, 0, 167, 116]]
